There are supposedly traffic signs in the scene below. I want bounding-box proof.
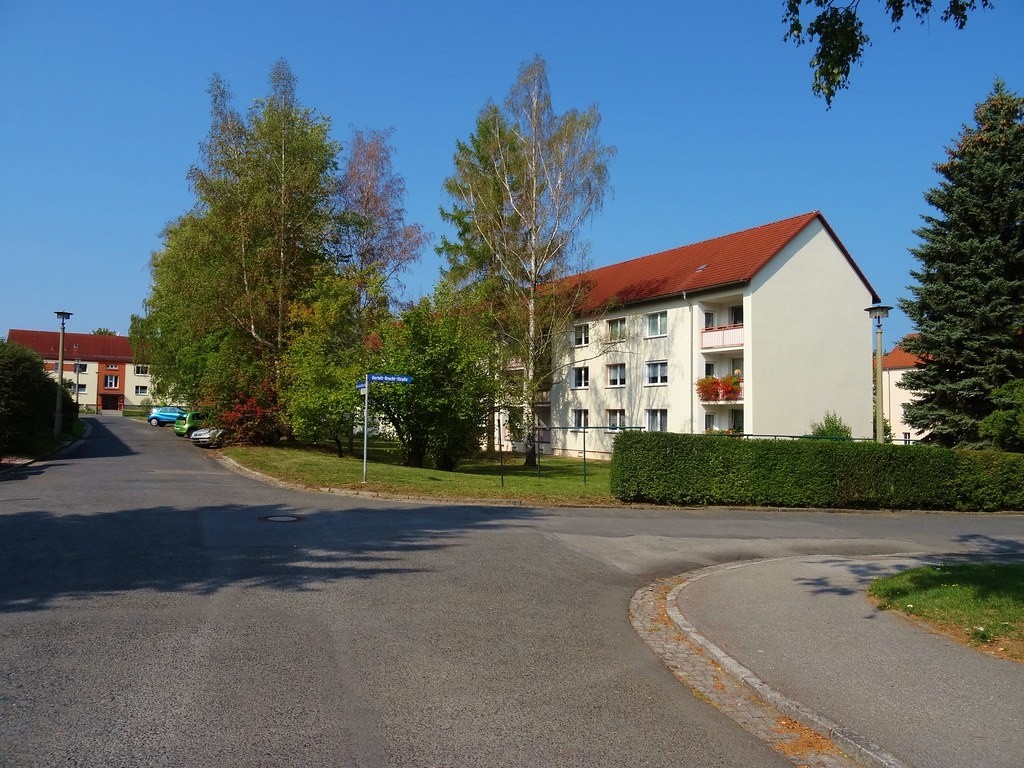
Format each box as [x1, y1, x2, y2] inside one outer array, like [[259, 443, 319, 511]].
[[355, 382, 366, 389], [368, 373, 414, 384]]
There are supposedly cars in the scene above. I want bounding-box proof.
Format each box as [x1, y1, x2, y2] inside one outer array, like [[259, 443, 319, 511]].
[[147, 406, 189, 427], [190, 427, 230, 448], [172, 411, 203, 438]]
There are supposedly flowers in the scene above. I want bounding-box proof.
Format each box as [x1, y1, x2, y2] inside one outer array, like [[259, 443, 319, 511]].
[[693, 370, 742, 401], [703, 427, 742, 439]]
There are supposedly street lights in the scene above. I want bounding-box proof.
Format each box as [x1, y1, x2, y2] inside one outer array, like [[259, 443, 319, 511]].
[[52, 311, 74, 441]]
